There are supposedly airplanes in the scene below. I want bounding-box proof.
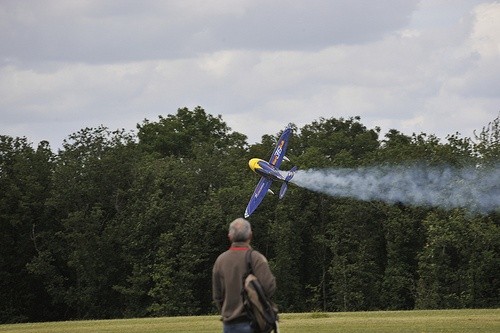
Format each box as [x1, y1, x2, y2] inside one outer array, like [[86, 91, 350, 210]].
[[242, 128, 298, 218]]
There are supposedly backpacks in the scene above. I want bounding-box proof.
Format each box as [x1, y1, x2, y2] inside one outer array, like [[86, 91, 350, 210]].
[[241, 249, 280, 333]]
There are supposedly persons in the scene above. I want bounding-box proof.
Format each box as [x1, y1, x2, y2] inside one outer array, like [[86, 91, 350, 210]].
[[212, 218, 277, 333]]
